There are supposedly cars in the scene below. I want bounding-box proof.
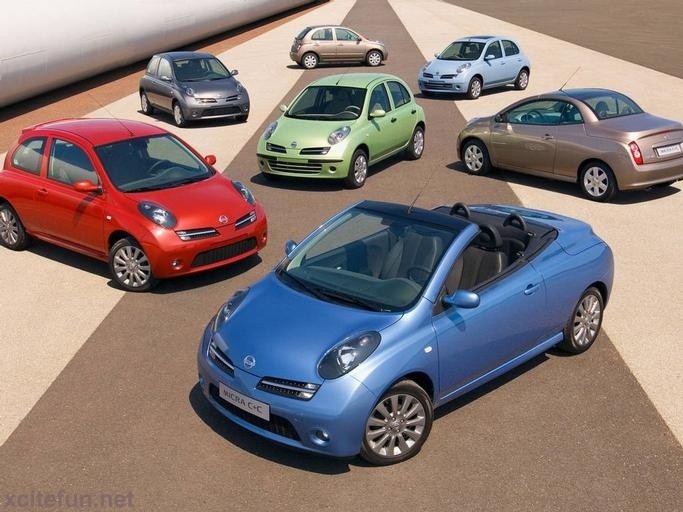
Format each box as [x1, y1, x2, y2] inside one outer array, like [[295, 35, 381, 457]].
[[456, 88, 683, 203], [138, 51, 250, 128], [417, 34, 532, 100], [255, 72, 427, 189], [0, 117, 269, 293], [196, 198, 615, 469], [289, 25, 388, 69]]
